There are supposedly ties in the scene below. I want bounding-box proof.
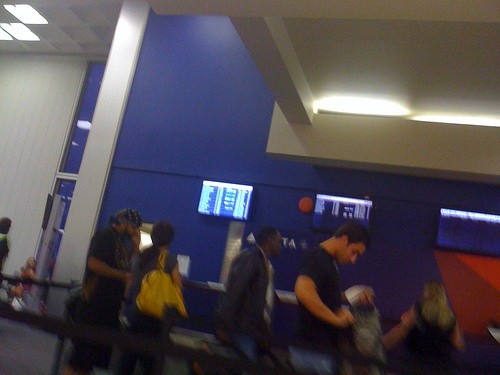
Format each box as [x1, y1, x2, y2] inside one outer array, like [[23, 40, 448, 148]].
[[262, 262, 275, 323]]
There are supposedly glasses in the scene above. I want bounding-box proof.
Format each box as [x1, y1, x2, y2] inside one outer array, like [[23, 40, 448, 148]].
[[129, 221, 141, 230]]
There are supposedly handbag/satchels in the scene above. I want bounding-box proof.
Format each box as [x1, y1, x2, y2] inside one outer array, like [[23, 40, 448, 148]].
[[135, 247, 190, 320], [353, 303, 384, 362]]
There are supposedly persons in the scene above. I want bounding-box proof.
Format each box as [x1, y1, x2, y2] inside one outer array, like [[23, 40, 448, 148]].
[[288, 223, 371, 375], [115, 221, 184, 375], [1, 217, 12, 275], [382, 280, 466, 375], [336, 288, 381, 375], [9, 259, 50, 299], [62, 206, 141, 375], [213, 226, 284, 375]]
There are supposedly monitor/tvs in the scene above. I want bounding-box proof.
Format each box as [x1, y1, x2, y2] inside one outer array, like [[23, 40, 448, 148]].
[[435, 206, 500, 258], [197, 178, 255, 222], [310, 192, 375, 235]]
